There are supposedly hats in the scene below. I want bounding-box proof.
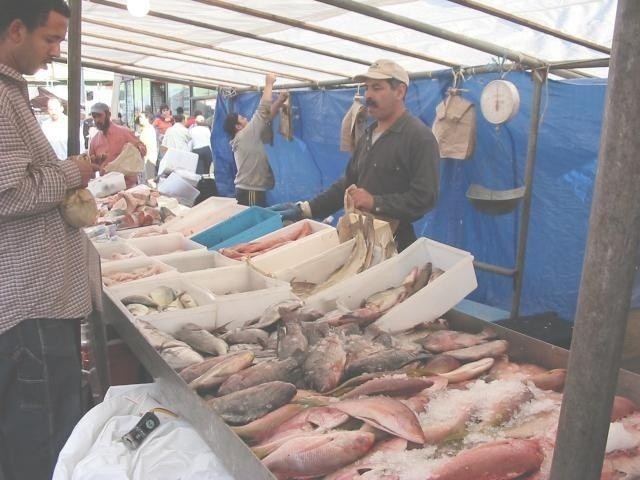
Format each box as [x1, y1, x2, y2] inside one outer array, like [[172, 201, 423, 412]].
[[88, 102, 109, 116], [352, 59, 409, 89], [195, 115, 204, 123]]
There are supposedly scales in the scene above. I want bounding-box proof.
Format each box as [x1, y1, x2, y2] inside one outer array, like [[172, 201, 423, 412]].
[[465, 79, 526, 216]]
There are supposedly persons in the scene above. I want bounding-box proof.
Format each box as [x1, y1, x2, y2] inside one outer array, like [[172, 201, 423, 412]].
[[223, 75, 289, 207], [0, 1, 100, 480], [267, 59, 440, 254], [41, 97, 85, 163], [81, 103, 213, 189]]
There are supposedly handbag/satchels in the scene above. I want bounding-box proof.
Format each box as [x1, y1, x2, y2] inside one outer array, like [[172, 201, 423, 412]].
[[60, 188, 97, 228], [338, 208, 400, 246], [195, 175, 218, 205]]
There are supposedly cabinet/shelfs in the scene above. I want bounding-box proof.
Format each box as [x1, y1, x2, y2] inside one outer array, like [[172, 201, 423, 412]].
[[94, 288, 639, 480]]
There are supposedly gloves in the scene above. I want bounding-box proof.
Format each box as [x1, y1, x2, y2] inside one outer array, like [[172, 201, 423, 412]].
[[266, 201, 312, 220]]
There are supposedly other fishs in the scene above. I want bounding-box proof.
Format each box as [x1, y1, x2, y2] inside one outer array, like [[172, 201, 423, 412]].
[[136, 299, 508, 479]]
